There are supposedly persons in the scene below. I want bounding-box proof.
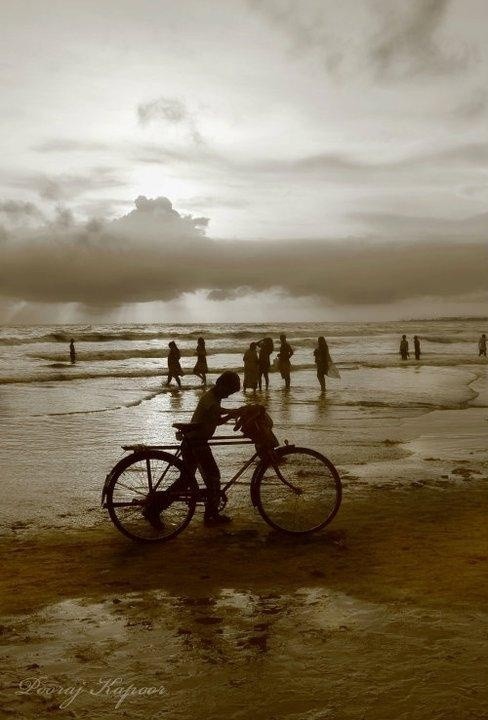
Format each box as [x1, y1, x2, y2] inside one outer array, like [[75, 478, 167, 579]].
[[142, 369, 250, 530], [242, 342, 260, 395], [162, 340, 184, 387], [399, 335, 410, 360], [68, 337, 76, 362], [193, 337, 209, 385], [312, 335, 331, 392], [478, 333, 488, 357], [256, 338, 275, 390], [412, 336, 422, 360], [276, 335, 295, 388]]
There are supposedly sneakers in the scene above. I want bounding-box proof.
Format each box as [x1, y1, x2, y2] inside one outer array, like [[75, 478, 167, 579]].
[[210, 515, 232, 522], [143, 511, 164, 529]]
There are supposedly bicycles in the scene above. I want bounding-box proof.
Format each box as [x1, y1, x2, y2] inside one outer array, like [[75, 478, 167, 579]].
[[99, 404, 343, 541]]
